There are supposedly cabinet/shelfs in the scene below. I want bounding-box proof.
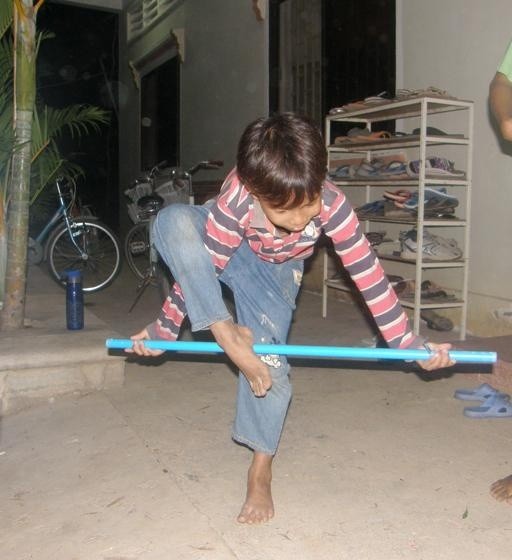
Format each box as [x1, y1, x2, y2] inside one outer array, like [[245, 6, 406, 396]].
[[321, 92, 477, 343]]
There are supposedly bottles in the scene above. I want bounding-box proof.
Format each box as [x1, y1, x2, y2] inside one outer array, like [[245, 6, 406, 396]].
[[62, 270, 85, 330]]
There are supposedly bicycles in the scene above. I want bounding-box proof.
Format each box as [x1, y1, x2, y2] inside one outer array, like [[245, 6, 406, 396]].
[[124, 160, 223, 315], [27, 176, 123, 294]]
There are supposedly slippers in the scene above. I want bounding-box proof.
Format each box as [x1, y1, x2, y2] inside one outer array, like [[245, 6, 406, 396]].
[[388, 275, 457, 302], [353, 186, 459, 218], [421, 310, 454, 331], [328, 126, 466, 178], [464, 395, 511, 419], [455, 383, 511, 402]]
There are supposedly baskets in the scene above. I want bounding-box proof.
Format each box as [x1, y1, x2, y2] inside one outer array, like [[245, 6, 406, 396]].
[[155, 179, 188, 206], [126, 182, 154, 224]]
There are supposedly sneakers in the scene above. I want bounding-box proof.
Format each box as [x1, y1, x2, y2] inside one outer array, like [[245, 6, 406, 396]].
[[399, 228, 462, 261]]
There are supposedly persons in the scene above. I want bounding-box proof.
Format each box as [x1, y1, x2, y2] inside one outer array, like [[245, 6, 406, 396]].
[[124, 114, 456, 526], [488, 38, 512, 504]]
